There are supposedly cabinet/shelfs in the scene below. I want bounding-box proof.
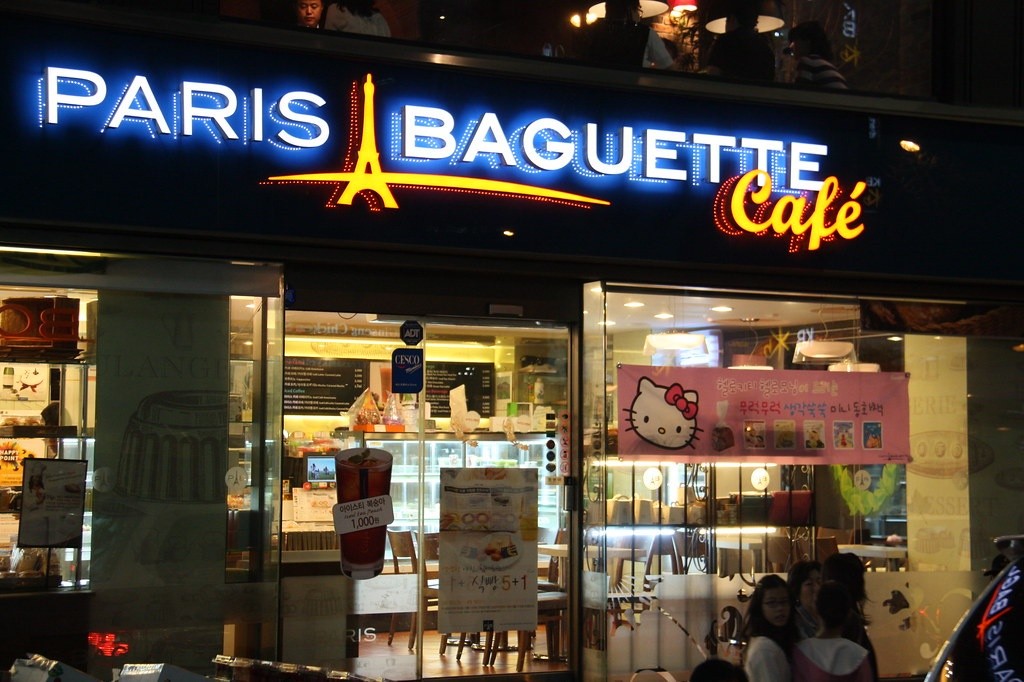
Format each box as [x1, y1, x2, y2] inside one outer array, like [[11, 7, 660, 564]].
[[591, 429, 815, 682], [332, 430, 570, 633], [0, 279, 267, 657]]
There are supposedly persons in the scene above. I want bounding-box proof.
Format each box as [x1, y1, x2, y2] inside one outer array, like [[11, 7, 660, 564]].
[[22, 472, 56, 513], [737, 572, 798, 682], [290, 0, 323, 30], [791, 583, 876, 680], [311, 463, 331, 480], [822, 550, 880, 681], [703, 619, 718, 659], [322, 0, 392, 38], [786, 558, 828, 643], [787, 19, 851, 90], [693, 0, 776, 80], [572, 0, 688, 73]]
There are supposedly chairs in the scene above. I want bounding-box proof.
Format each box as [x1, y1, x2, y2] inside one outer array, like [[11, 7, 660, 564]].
[[386, 527, 568, 671], [759, 537, 842, 573], [607, 531, 707, 628]]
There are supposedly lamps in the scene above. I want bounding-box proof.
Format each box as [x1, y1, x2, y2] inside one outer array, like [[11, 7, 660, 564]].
[[792, 302, 858, 364], [644, 296, 709, 356], [828, 308, 880, 372], [726, 321, 774, 370]]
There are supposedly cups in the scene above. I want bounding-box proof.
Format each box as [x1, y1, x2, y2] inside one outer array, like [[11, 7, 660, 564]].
[[611, 500, 632, 524], [670, 507, 685, 524], [724, 504, 738, 524], [0, 555, 10, 570], [638, 499, 655, 524], [333, 447, 394, 581], [770, 489, 811, 526]]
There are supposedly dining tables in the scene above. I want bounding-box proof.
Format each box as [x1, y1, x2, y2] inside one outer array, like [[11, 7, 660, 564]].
[[749, 543, 908, 573], [535, 545, 647, 657]]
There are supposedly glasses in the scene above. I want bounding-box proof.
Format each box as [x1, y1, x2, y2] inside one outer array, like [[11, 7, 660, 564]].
[[763, 600, 791, 608]]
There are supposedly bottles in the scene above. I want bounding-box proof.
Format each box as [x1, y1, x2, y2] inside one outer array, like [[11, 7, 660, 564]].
[[534, 378, 544, 404]]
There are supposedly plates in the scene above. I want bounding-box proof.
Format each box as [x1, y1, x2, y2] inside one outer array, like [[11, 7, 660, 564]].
[[477, 532, 522, 569]]
